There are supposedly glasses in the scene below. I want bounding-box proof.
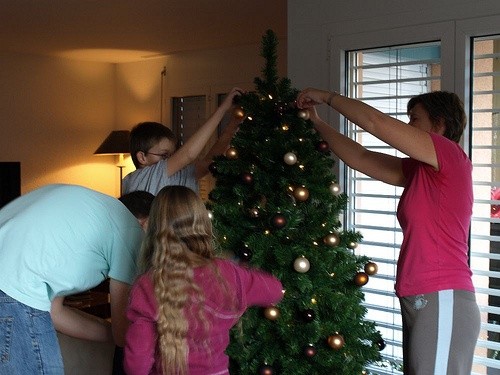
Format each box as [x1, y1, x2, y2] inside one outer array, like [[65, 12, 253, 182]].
[[148, 152, 172, 160]]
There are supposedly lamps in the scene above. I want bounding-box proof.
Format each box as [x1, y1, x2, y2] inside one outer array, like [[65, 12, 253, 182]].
[[93, 131, 132, 198]]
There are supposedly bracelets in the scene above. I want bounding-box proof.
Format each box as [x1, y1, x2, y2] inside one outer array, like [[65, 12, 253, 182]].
[[327, 89, 340, 106]]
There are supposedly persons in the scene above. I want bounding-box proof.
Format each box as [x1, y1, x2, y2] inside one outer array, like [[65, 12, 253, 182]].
[[297, 88, 480, 375], [112, 86, 243, 375], [0, 184, 154, 375], [123, 185, 284, 375]]
